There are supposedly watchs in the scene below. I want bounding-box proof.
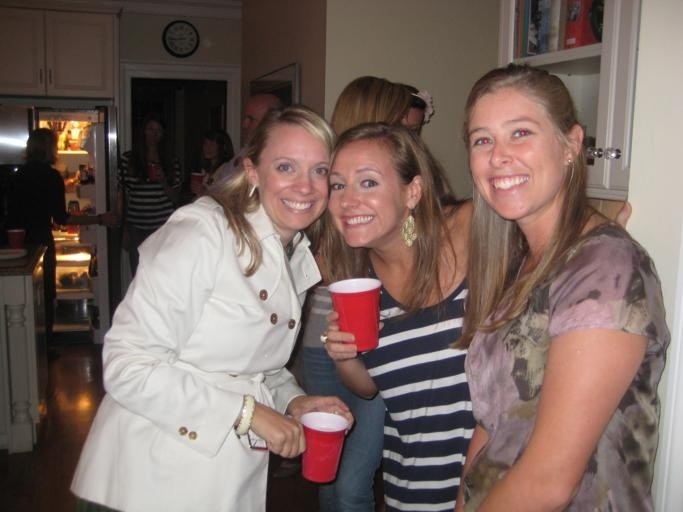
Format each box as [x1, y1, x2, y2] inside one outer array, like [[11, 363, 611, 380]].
[[234, 395, 255, 439]]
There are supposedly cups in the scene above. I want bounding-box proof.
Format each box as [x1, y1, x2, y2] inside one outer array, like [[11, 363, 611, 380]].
[[148, 162, 164, 183], [8, 229, 25, 248], [327, 278, 382, 353], [300, 412, 349, 484], [190, 172, 205, 195]]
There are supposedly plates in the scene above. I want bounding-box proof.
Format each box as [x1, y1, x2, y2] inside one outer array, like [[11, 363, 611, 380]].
[[0, 249, 28, 261]]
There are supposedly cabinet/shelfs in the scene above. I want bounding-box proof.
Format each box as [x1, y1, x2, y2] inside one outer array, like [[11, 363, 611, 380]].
[[500, 1, 642, 202], [0, 1, 120, 99]]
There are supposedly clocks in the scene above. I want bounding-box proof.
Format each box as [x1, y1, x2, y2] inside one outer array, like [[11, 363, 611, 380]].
[[161, 18, 200, 58]]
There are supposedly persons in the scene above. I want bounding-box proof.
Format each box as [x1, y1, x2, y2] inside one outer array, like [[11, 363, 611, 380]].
[[8, 128, 117, 352], [297, 76, 435, 512], [330, 121, 476, 512], [199, 130, 235, 194], [115, 115, 181, 280], [214, 90, 295, 185], [70, 100, 337, 512], [448, 59, 670, 512]]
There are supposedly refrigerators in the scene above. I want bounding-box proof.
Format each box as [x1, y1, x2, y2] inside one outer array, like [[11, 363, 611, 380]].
[[26, 106, 112, 345]]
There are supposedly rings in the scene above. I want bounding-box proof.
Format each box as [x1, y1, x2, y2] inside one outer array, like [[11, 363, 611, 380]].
[[320, 334, 328, 343]]
